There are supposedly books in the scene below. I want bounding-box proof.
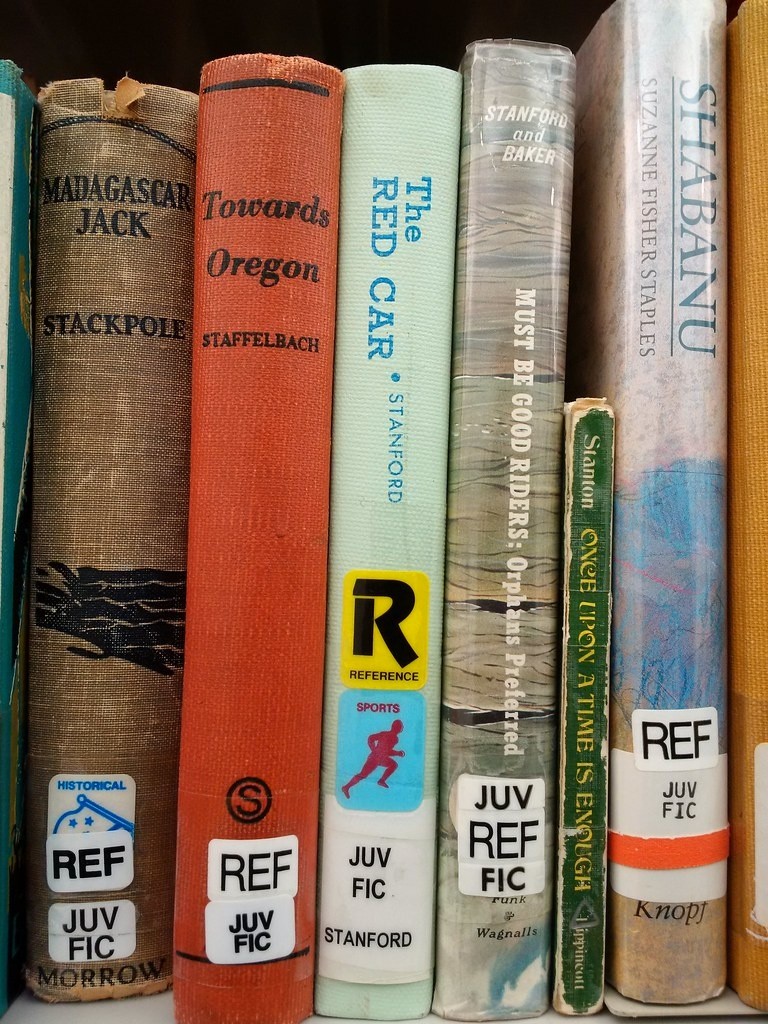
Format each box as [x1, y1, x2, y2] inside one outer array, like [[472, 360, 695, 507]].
[[432, 37, 577, 1021], [566, 1, 728, 1004], [173, 56, 346, 1024], [315, 64, 464, 1020], [0, 59, 199, 1024], [552, 397, 617, 1018], [732, 1, 768, 1012]]
